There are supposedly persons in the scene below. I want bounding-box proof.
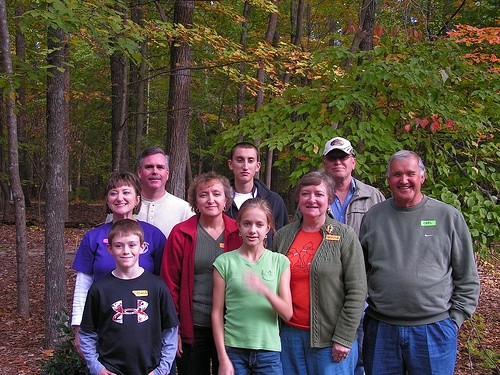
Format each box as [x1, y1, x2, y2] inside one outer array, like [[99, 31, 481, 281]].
[[272, 171, 367, 375], [70, 170, 167, 361], [222, 143, 289, 250], [161, 171, 243, 375], [211, 197, 294, 375], [359, 151, 480, 375], [292, 137, 386, 375], [79, 219, 178, 375], [105, 147, 198, 240]]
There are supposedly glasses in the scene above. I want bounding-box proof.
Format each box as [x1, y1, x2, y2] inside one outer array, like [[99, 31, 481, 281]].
[[324, 154, 353, 162]]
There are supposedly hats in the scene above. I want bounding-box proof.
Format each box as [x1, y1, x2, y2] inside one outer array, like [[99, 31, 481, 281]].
[[322, 137, 353, 155]]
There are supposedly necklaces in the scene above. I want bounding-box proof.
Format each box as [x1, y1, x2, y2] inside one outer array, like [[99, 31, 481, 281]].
[[251, 184, 257, 198]]
